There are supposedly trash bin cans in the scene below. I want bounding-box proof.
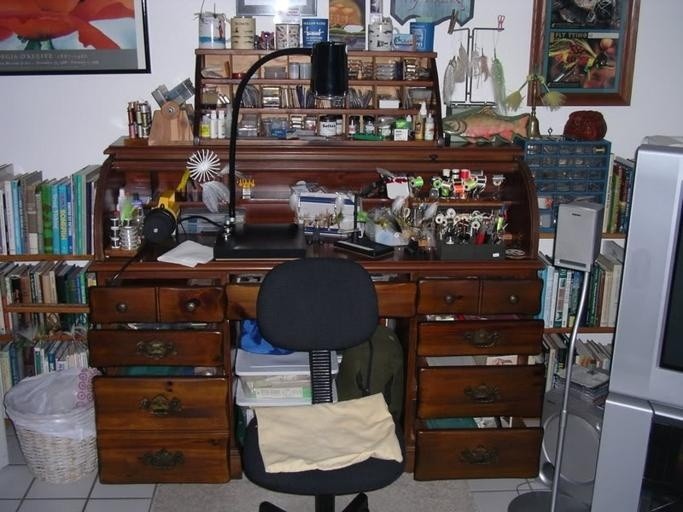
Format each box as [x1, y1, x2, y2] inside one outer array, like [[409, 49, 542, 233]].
[[4, 368, 101, 485]]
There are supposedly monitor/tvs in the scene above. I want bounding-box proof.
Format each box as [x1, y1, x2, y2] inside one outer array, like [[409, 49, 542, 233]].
[[591, 393, 683, 512], [608, 144, 683, 410]]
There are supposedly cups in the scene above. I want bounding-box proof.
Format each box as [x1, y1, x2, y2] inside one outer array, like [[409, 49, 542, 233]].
[[199, 17, 434, 55]]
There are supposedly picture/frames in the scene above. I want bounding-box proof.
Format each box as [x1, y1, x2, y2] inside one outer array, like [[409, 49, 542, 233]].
[[0, 0, 152, 76], [527, 0, 640, 106]]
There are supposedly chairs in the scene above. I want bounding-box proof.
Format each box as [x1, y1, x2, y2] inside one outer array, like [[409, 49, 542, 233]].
[[234, 258, 408, 512]]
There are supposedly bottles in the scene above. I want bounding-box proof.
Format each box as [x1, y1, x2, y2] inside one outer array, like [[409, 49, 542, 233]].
[[407, 99, 435, 142], [317, 115, 375, 139], [288, 62, 311, 80], [114, 187, 144, 251], [197, 108, 231, 140]]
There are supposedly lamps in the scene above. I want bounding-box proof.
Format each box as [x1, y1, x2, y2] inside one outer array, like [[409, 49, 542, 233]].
[[230, 42, 348, 218]]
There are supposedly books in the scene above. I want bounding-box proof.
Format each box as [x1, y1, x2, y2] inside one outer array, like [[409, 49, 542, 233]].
[[539, 154, 636, 406], [0, 162, 102, 418]]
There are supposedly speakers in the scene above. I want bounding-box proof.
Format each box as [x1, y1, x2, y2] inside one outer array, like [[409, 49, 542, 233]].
[[539, 387, 604, 507], [553, 198, 604, 273]]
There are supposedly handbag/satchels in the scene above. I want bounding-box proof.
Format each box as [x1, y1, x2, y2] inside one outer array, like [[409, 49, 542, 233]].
[[335, 326, 404, 421]]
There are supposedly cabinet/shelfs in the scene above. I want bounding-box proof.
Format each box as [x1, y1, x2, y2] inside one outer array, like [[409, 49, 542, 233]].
[[194, 48, 443, 147], [0, 255, 100, 342], [84, 136, 545, 484]]
